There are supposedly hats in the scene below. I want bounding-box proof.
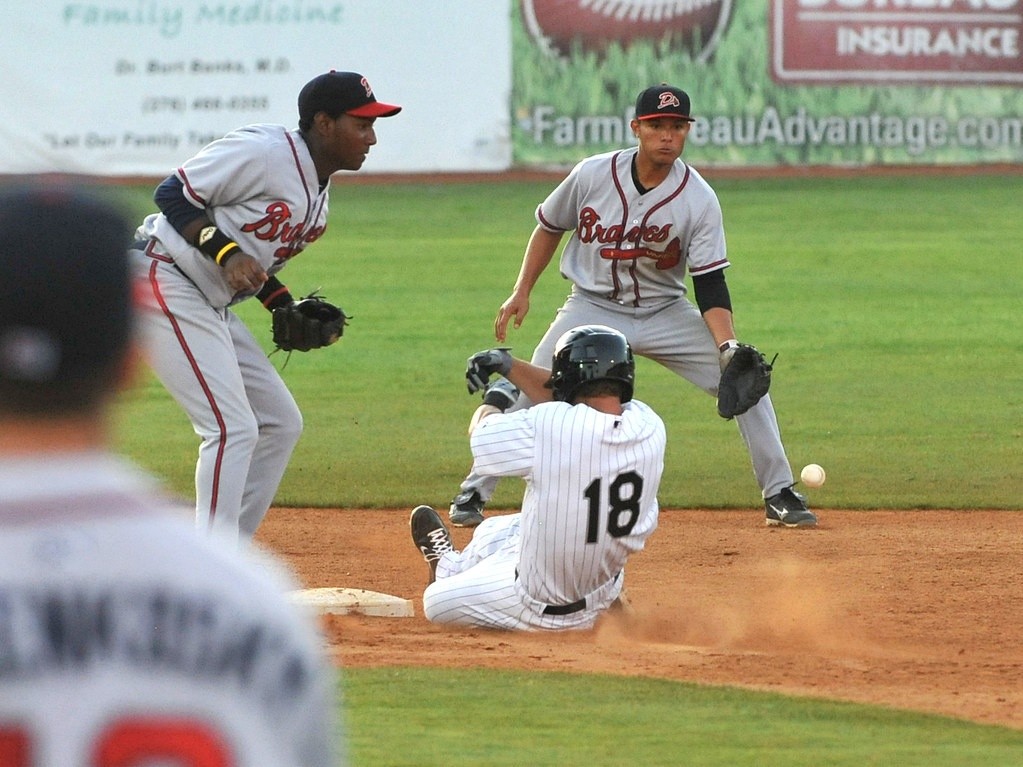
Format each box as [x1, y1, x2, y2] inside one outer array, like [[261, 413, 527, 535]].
[[299, 70, 402, 121], [635, 83, 696, 123], [3, 175, 137, 407]]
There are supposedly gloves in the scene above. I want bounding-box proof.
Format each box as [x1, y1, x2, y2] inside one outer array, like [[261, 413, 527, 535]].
[[466, 348, 512, 395], [482, 381, 521, 413]]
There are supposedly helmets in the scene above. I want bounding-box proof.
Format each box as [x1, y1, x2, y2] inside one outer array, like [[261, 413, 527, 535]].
[[544, 325, 636, 404]]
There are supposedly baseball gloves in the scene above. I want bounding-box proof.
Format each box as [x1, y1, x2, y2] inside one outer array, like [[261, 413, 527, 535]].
[[271, 297, 346, 354], [717, 345, 771, 419]]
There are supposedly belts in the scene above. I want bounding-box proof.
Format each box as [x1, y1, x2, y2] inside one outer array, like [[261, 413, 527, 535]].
[[514, 568, 621, 615]]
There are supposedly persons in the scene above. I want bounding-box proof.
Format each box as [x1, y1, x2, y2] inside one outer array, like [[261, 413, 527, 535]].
[[127, 70, 402, 542], [447, 83, 817, 528], [409, 324, 667, 633], [1, 179, 341, 767]]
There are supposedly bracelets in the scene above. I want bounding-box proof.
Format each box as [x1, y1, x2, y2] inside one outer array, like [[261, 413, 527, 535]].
[[216, 242, 238, 266], [264, 287, 288, 307]]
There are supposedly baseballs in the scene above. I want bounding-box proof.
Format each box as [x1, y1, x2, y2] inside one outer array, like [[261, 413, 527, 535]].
[[800, 463, 826, 489]]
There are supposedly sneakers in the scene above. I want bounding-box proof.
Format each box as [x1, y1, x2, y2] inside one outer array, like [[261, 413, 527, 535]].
[[448, 489, 486, 527], [764, 481, 816, 527], [409, 504, 456, 584]]
